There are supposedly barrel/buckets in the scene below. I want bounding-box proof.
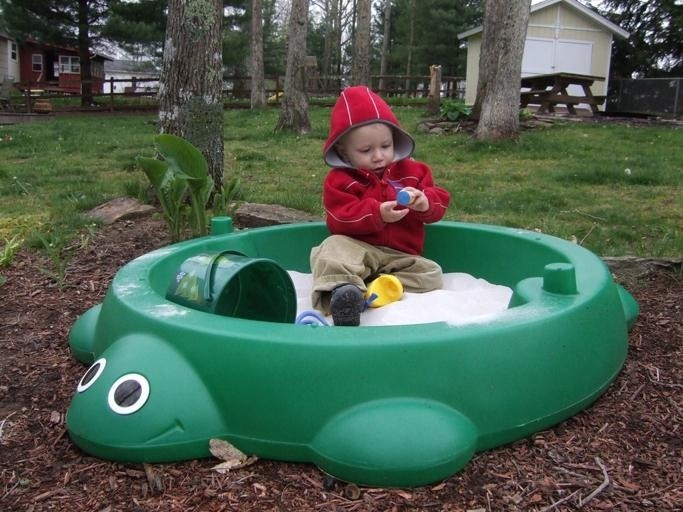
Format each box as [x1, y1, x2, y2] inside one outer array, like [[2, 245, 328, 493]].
[[164, 248, 297, 325]]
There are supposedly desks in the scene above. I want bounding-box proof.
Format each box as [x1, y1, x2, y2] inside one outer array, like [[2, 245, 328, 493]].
[[521, 72, 606, 116]]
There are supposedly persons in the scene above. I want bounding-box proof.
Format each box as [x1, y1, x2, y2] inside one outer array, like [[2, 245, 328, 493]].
[[310, 83, 453, 327]]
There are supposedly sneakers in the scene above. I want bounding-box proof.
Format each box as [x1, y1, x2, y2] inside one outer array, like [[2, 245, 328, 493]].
[[329, 284, 362, 326]]
